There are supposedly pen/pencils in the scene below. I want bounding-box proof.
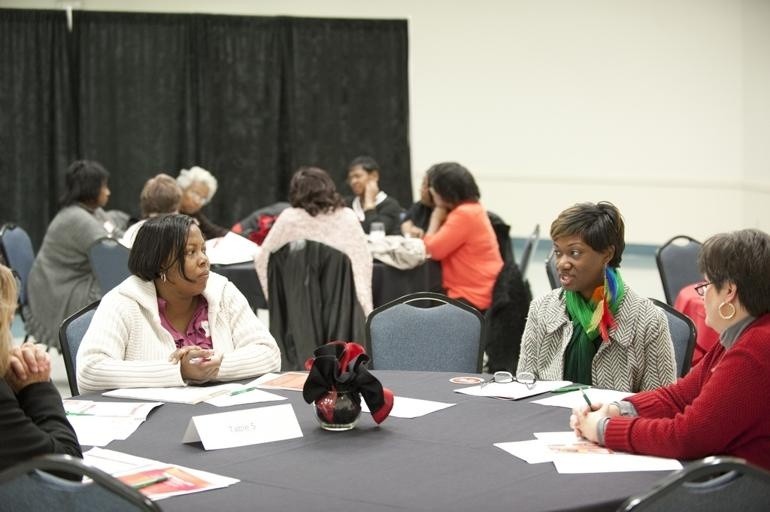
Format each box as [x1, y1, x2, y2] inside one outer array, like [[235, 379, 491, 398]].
[[62, 411, 95, 417], [188, 357, 209, 366], [230, 385, 257, 394], [126, 476, 168, 492], [578, 387, 599, 410]]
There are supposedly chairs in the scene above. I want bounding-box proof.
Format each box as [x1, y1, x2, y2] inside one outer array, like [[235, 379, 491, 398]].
[[654, 235, 704, 306], [545, 245, 562, 289], [266, 238, 367, 371], [0, 223, 50, 353], [617, 456, 767, 511], [59, 300, 102, 397], [0, 454, 163, 511], [518, 224, 540, 278], [87, 237, 133, 295], [649, 297, 698, 379], [365, 291, 486, 373]]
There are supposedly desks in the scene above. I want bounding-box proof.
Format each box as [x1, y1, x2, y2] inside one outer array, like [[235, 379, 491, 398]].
[[1, 369, 707, 511], [210, 260, 443, 317]]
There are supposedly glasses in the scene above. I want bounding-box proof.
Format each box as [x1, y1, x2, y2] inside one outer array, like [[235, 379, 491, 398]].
[[693, 278, 725, 296], [187, 190, 207, 206], [480, 371, 537, 390]]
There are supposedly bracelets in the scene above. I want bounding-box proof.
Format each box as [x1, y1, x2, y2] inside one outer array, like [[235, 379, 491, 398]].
[[596, 417, 609, 449]]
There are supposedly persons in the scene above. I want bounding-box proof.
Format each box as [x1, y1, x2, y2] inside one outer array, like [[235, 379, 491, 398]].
[[423, 161, 505, 317], [116, 172, 183, 250], [252, 167, 374, 323], [75, 213, 282, 396], [23, 159, 126, 356], [515, 200, 677, 394], [340, 155, 401, 235], [399, 163, 435, 239], [568, 228, 770, 471], [175, 165, 253, 241], [0, 264, 78, 443]]
